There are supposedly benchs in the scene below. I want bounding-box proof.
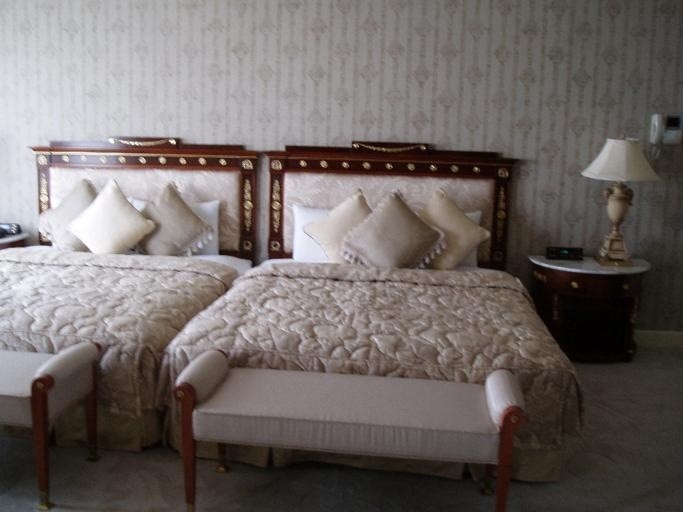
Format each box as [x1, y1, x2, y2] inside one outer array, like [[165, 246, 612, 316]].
[[174, 349, 528, 512]]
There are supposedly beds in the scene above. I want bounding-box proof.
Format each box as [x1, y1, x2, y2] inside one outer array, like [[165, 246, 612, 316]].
[[161, 140, 574, 481], [0, 137, 260, 452]]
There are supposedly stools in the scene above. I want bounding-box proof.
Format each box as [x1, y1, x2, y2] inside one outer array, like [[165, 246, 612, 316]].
[[0, 341, 101, 508]]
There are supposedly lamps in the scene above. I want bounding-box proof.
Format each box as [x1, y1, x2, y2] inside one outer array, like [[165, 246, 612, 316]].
[[581, 138, 662, 265]]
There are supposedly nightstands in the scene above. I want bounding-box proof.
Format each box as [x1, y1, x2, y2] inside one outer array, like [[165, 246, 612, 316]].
[[0, 222, 28, 250], [529, 255, 650, 363]]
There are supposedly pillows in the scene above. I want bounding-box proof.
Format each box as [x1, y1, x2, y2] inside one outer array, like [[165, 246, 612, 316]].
[[417, 189, 490, 270], [291, 203, 343, 262], [140, 183, 211, 258], [342, 191, 445, 270], [69, 181, 157, 254], [452, 210, 482, 271], [185, 200, 221, 256], [35, 178, 98, 252], [303, 188, 375, 265]]
